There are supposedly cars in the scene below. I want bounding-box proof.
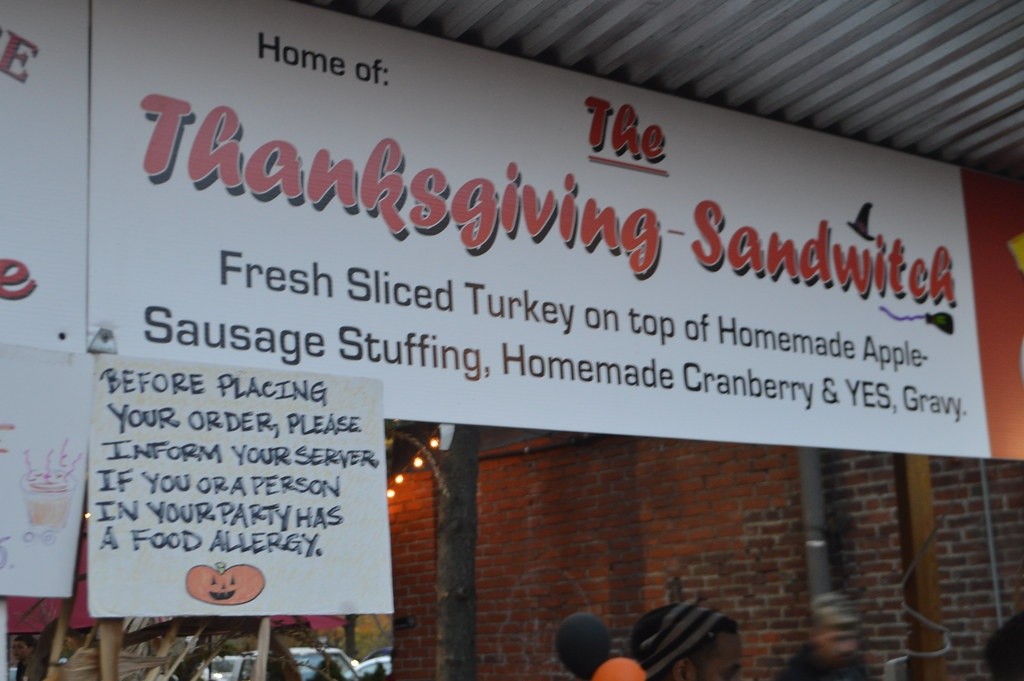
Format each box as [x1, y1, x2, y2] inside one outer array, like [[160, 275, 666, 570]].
[[232, 648, 360, 681], [347, 648, 393, 679], [203, 655, 240, 681]]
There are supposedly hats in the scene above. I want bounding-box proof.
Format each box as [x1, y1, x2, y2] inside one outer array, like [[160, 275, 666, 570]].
[[630, 603, 728, 681], [810, 592, 860, 625]]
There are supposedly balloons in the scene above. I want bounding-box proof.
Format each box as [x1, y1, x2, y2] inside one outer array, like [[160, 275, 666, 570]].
[[594, 658, 646, 681], [555, 613, 611, 677]]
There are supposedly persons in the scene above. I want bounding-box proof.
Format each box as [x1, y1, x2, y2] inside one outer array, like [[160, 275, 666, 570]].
[[781, 591, 869, 681], [629, 604, 739, 681]]
